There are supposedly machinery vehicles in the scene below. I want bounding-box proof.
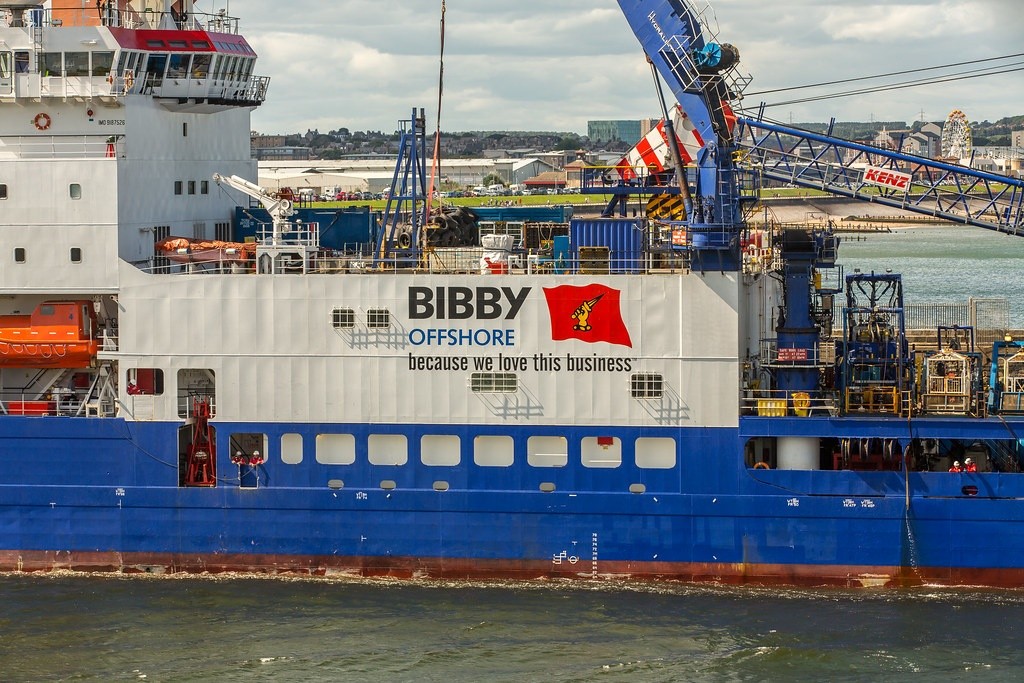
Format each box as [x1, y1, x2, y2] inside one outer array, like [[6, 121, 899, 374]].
[[212, 173, 320, 257]]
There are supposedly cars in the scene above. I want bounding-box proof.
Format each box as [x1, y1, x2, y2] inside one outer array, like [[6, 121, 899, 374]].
[[361, 192, 372, 200], [440, 192, 448, 198], [530, 187, 583, 195], [522, 190, 531, 196], [385, 193, 397, 199], [915, 180, 967, 186], [513, 190, 523, 196], [787, 183, 800, 189]]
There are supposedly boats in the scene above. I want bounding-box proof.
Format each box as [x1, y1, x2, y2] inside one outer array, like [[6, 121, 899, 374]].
[[0, 0, 1024, 592]]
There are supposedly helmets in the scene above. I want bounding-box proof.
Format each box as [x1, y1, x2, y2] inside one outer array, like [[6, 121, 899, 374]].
[[130, 379, 137, 386], [965, 458, 972, 465], [954, 461, 960, 466], [253, 451, 260, 456], [236, 451, 242, 456]]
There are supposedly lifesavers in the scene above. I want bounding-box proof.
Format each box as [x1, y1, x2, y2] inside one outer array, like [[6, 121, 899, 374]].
[[398, 205, 480, 248], [34, 113, 51, 130]]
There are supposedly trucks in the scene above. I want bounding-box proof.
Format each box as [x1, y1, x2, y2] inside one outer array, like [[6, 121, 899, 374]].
[[472, 187, 486, 197], [325, 187, 341, 201], [489, 184, 503, 196]]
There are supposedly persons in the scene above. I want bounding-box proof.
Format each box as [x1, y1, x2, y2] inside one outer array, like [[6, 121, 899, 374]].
[[249, 451, 263, 466], [127, 379, 141, 395], [231, 451, 245, 465], [488, 197, 522, 207], [949, 458, 977, 472]]
